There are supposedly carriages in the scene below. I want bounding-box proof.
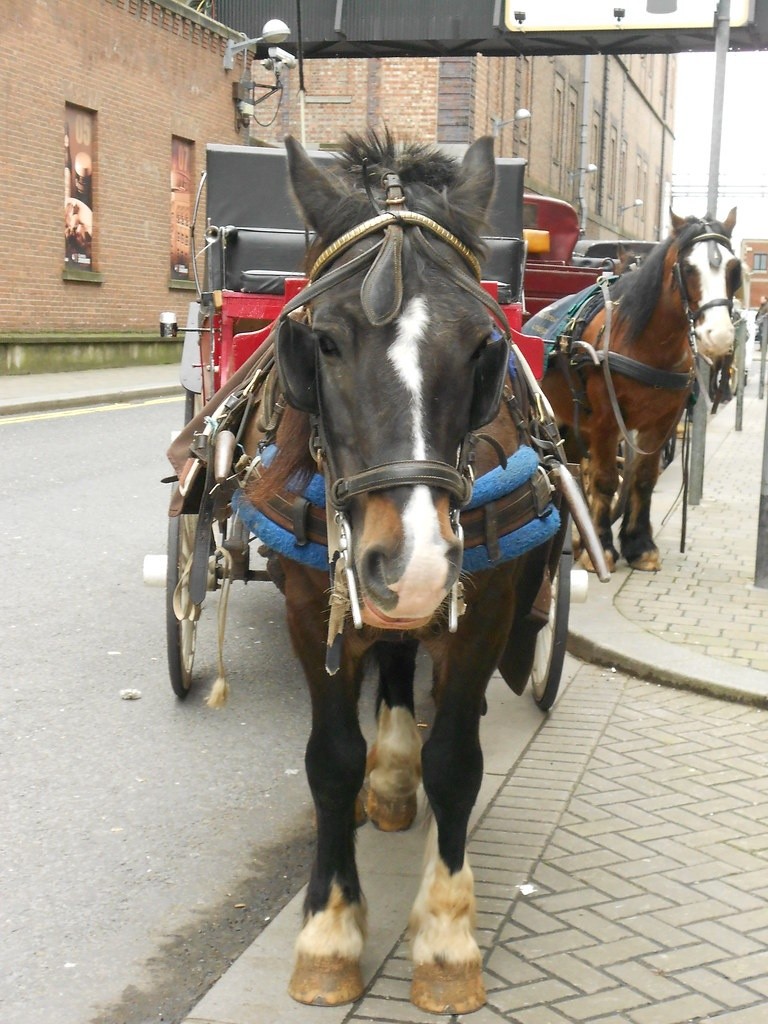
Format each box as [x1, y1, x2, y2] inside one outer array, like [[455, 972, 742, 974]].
[[522, 192, 748, 572], [165, 121, 613, 1017]]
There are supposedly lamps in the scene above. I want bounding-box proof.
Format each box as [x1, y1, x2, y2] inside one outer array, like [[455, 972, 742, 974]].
[[568, 163, 598, 186], [223, 18, 291, 70], [490, 107, 532, 137], [620, 198, 644, 214]]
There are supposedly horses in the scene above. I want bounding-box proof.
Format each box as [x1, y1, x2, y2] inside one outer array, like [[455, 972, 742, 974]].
[[523, 205, 738, 572], [241, 130, 574, 1016]]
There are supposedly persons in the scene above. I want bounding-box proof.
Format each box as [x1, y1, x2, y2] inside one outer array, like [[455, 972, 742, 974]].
[[755, 296, 768, 352]]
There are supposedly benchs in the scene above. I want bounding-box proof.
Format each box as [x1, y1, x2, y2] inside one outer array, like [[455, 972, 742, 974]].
[[204, 145, 529, 303], [584, 242, 660, 264]]
